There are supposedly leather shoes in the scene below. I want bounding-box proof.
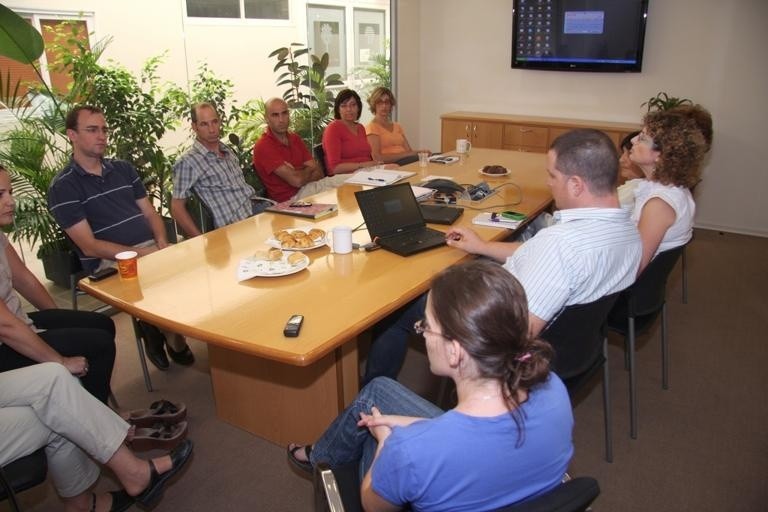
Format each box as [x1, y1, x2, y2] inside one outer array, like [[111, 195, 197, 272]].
[[91, 491, 134, 512], [160, 330, 195, 365], [134, 438, 195, 512], [137, 320, 170, 371]]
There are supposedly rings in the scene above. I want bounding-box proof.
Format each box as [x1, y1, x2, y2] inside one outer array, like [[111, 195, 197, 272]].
[[84, 367, 88, 372]]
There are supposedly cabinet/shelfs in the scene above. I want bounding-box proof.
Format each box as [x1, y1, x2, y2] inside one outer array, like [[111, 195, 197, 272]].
[[549, 125, 621, 153], [502, 122, 549, 152], [621, 131, 644, 143], [440, 118, 502, 153]]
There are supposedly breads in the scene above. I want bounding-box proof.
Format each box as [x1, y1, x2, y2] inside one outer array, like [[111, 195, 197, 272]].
[[288, 253, 304, 267], [255, 248, 282, 260], [274, 229, 325, 250], [483, 165, 506, 174]]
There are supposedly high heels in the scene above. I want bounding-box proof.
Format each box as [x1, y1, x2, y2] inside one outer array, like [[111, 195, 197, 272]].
[[130, 421, 188, 451], [129, 400, 187, 428]]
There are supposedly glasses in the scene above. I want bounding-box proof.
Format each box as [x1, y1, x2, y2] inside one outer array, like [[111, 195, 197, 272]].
[[414, 319, 451, 341]]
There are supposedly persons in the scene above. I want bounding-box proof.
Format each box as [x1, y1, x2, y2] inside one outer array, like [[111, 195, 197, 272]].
[[322, 89, 384, 178], [47, 104, 195, 370], [359, 129, 643, 391], [254, 99, 324, 204], [618, 130, 644, 180], [1, 361, 194, 511], [512, 105, 713, 284], [365, 87, 432, 164], [170, 102, 278, 239], [0, 166, 136, 445], [287, 261, 574, 512]]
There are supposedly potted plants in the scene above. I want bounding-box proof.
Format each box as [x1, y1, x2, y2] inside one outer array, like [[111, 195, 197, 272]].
[[0, 130, 101, 289]]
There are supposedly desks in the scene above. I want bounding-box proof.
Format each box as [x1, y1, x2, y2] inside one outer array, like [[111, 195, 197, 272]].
[[72, 143, 588, 452]]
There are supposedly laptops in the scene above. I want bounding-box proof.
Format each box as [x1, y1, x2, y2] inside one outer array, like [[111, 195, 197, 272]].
[[354, 181, 461, 258]]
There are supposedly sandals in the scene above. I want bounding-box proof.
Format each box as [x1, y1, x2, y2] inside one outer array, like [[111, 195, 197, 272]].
[[286, 442, 313, 473]]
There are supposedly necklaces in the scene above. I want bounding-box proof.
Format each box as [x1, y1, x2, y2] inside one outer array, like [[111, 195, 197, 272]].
[[458, 395, 503, 403]]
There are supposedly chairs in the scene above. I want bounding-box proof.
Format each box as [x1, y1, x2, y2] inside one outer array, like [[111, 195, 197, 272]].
[[546, 290, 624, 464], [315, 464, 600, 511], [593, 243, 688, 441], [56, 215, 178, 395]]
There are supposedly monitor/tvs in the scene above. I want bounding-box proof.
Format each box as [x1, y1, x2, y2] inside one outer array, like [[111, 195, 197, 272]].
[[511, 0, 649, 73]]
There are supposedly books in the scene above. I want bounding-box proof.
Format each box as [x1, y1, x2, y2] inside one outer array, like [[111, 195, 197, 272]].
[[265, 200, 337, 220], [472, 212, 523, 230], [427, 154, 459, 164]]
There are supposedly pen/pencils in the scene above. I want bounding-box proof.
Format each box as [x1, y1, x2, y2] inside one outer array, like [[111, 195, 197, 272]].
[[368, 178, 385, 181], [489, 218, 517, 222]]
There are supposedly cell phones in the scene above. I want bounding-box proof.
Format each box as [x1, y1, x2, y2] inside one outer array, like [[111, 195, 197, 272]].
[[289, 201, 312, 207], [283, 314, 304, 337], [502, 210, 527, 221], [87, 267, 118, 282]]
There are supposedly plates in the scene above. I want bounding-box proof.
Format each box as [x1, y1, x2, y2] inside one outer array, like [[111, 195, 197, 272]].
[[270, 228, 327, 252], [252, 249, 309, 277], [479, 167, 511, 176]]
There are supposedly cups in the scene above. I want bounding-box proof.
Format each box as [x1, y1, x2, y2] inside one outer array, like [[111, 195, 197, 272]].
[[418, 152, 430, 167], [331, 227, 353, 254], [114, 250, 138, 281], [456, 138, 471, 154]]
